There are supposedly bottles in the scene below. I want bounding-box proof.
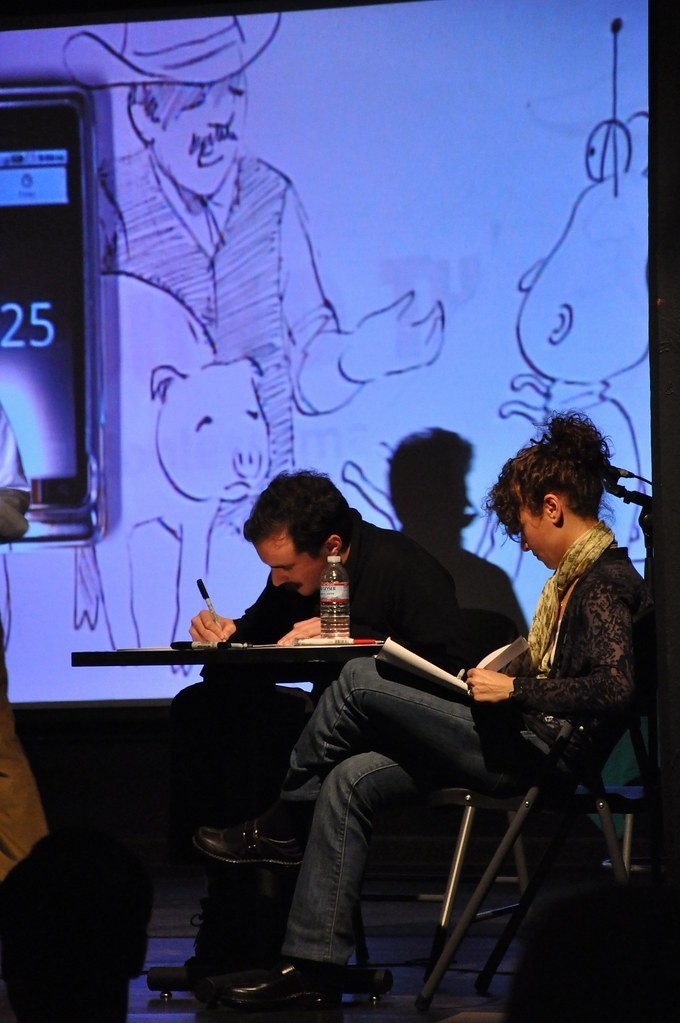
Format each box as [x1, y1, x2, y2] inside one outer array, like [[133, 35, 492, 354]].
[[319, 556, 350, 641]]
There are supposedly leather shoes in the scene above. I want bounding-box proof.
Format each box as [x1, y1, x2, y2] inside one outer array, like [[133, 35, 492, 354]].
[[192, 818, 305, 874], [219, 962, 342, 1010]]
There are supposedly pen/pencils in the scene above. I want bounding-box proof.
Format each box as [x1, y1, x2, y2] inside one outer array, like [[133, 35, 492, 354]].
[[196, 578, 227, 642], [354, 638, 386, 644], [456, 669, 465, 679]]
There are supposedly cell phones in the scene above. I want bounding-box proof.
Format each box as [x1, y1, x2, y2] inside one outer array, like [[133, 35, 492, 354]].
[[0, 85, 106, 549]]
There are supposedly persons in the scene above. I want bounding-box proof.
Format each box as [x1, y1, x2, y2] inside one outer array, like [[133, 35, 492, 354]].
[[0, 818, 153, 1023], [0, 397, 51, 884], [497, 882, 680, 1023], [192, 414, 651, 1011], [160, 468, 468, 974]]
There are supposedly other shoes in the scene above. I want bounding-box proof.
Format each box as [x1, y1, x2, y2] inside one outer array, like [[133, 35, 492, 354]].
[[184, 897, 276, 976]]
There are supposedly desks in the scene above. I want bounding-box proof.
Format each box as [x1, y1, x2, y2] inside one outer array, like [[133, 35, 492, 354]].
[[62, 637, 403, 1010]]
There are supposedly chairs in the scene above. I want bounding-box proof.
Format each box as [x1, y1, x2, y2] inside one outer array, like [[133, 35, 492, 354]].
[[399, 592, 668, 1012]]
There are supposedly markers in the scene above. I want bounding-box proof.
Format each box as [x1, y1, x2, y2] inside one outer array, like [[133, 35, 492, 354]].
[[170, 641, 231, 649]]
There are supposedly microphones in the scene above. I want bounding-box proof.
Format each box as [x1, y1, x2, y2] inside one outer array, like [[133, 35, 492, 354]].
[[604, 466, 635, 478]]
